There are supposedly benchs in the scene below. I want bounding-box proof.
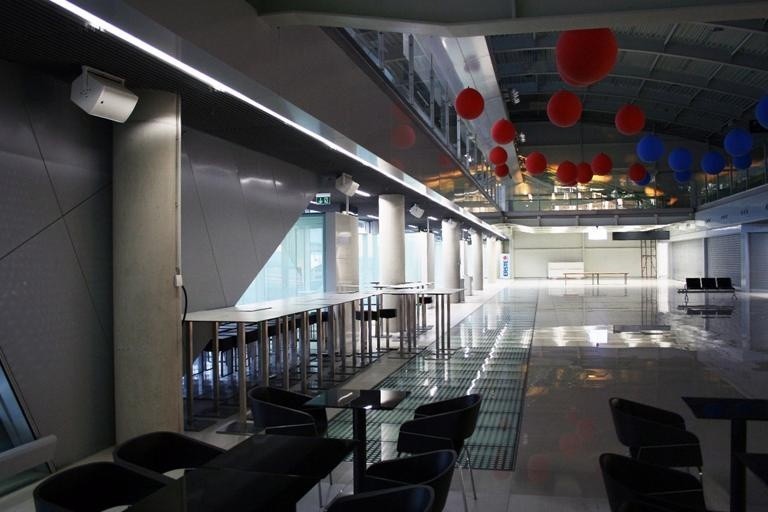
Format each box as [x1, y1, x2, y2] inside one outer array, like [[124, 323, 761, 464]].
[[565, 271, 629, 284]]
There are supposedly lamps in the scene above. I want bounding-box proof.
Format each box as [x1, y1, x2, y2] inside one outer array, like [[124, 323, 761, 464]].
[[70, 66, 139, 124], [410, 203, 425, 218], [588, 225, 608, 240], [336, 173, 359, 196]]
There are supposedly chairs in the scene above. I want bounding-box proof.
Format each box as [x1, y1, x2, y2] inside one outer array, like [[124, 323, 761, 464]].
[[599, 398, 767, 511], [679, 304, 734, 315], [686, 277, 735, 291]]
